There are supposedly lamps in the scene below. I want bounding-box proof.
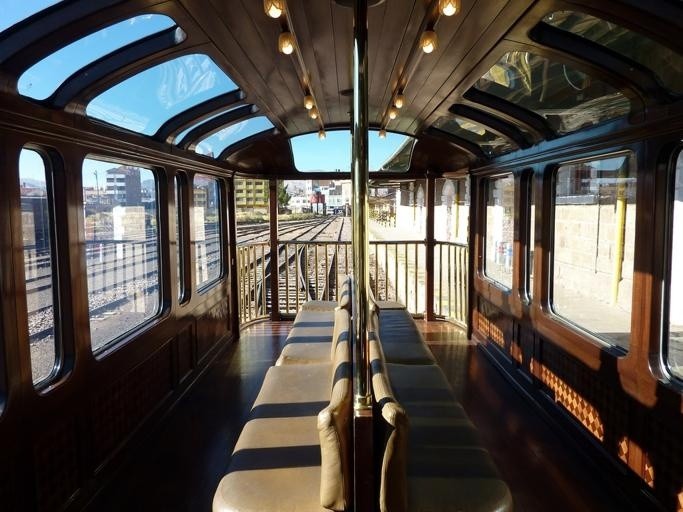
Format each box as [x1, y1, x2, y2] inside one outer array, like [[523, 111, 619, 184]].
[[263, 0, 460, 140]]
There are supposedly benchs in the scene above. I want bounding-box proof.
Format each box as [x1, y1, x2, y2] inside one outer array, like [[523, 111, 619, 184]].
[[212, 274, 350, 512], [367, 272, 513, 512]]
